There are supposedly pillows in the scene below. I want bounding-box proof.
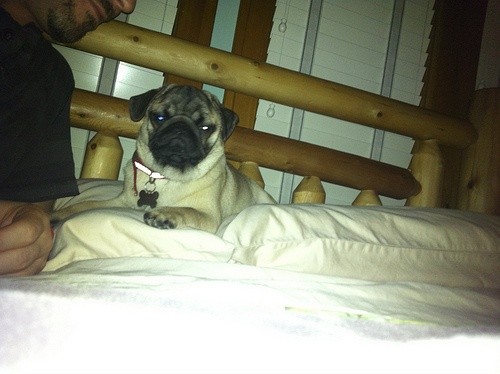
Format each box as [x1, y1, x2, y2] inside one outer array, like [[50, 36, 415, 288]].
[[41, 178, 235, 271], [217, 203, 500, 291]]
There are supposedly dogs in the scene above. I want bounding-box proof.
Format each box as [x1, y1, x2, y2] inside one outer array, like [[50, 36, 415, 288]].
[[42, 84, 278, 231]]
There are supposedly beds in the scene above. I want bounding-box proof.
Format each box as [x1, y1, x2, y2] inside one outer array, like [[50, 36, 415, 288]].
[[0, 19, 500, 374]]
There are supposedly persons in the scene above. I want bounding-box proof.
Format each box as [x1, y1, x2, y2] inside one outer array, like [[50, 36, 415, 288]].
[[0, 0, 137, 280]]
